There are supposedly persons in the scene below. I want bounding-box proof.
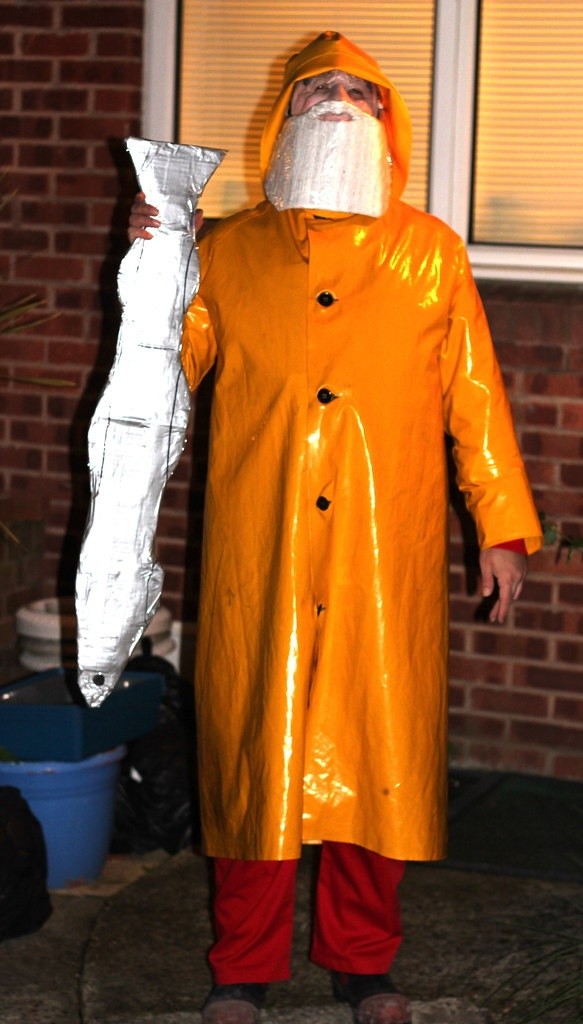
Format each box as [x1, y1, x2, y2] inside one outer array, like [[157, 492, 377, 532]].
[[127, 30, 544, 1024]]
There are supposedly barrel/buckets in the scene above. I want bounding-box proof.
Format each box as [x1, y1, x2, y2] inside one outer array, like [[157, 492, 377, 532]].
[[14, 595, 177, 676], [0, 701, 127, 894]]
